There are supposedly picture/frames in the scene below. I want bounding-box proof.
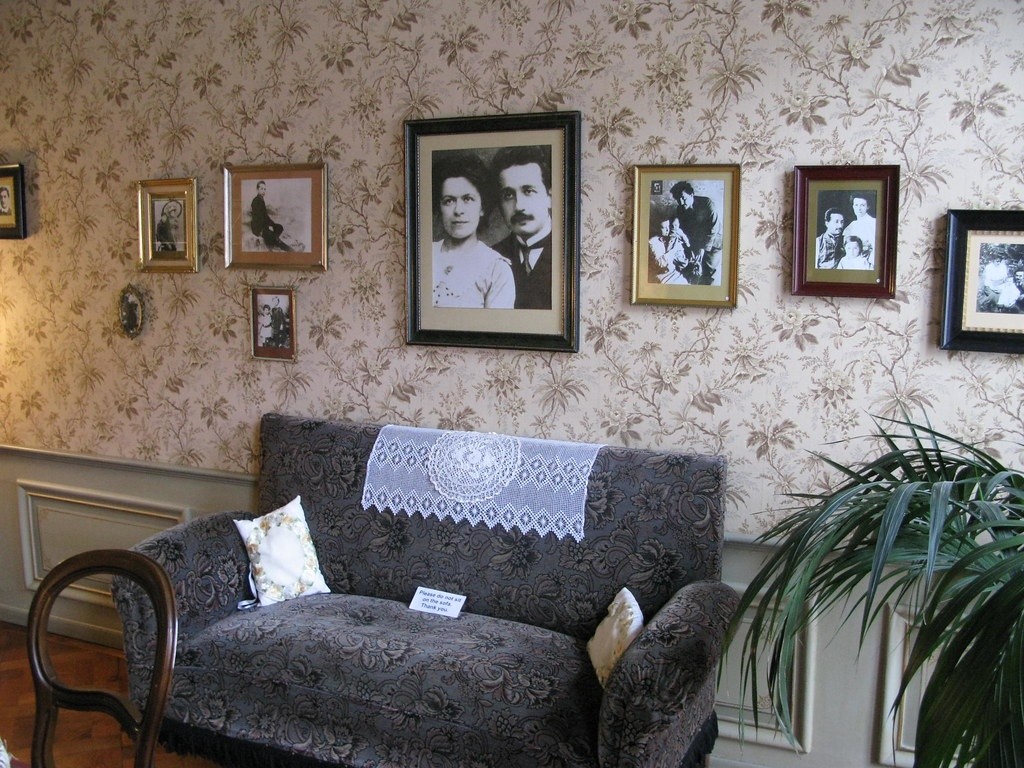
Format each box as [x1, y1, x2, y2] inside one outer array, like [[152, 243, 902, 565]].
[[116, 283, 146, 340], [224, 162, 329, 271], [630, 163, 741, 307], [939, 209, 1024, 355], [791, 164, 901, 299], [0, 163, 28, 239], [403, 111, 583, 353], [250, 286, 299, 363], [136, 178, 199, 273]]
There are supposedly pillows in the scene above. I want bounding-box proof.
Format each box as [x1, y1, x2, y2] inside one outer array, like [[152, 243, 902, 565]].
[[232, 495, 332, 608], [587, 588, 645, 691]]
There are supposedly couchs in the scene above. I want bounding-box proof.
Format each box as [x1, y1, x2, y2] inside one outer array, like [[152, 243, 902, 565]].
[[111, 412, 742, 768]]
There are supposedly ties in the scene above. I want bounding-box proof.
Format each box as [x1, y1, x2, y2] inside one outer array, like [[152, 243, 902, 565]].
[[828, 234, 839, 247], [518, 231, 552, 280]]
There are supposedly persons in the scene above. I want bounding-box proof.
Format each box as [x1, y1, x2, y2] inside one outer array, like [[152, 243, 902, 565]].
[[157, 212, 178, 250], [649, 181, 721, 285], [0, 187, 12, 216], [258, 296, 286, 347], [431, 146, 552, 310], [250, 181, 301, 254], [816, 190, 875, 269], [981, 252, 1024, 314]]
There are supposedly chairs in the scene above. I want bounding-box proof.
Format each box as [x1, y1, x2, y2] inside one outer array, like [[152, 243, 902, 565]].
[[28, 549, 178, 768]]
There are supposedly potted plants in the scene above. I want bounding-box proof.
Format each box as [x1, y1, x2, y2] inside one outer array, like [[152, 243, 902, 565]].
[[713, 396, 1024, 768]]
